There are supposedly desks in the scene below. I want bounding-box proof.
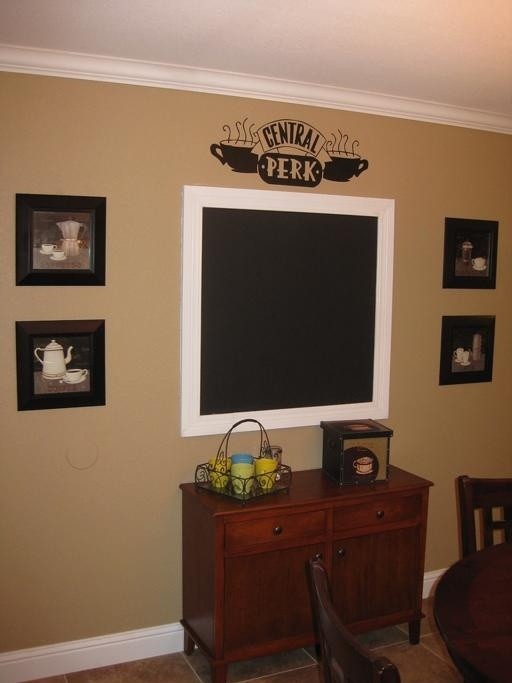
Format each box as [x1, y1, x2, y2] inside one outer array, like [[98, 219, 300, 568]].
[[430, 538, 512, 683]]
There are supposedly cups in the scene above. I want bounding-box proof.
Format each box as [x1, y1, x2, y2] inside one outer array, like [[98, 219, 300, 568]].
[[454, 347, 470, 366], [267, 446, 282, 482], [472, 256, 485, 268], [329, 155, 369, 181], [67, 367, 88, 381], [210, 138, 259, 169], [52, 249, 65, 258], [42, 243, 57, 251], [352, 456, 375, 471], [208, 453, 276, 492]]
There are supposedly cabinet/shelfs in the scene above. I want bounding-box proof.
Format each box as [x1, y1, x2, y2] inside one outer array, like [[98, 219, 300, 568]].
[[174, 457, 436, 683]]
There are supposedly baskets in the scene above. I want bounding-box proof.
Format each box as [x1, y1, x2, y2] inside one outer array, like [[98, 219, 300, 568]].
[[195, 419, 292, 505]]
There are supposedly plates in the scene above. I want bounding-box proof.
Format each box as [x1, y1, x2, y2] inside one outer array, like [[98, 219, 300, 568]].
[[64, 375, 86, 385], [50, 255, 68, 261], [356, 470, 373, 474], [40, 248, 53, 255], [472, 265, 486, 270]]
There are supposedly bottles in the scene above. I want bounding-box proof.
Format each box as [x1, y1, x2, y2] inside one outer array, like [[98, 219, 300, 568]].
[[472, 333, 482, 360], [462, 241, 472, 264]]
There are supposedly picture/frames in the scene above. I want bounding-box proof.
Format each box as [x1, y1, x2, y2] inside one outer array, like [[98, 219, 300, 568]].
[[176, 183, 396, 438], [14, 316, 108, 413], [437, 313, 495, 388], [14, 190, 109, 288], [441, 216, 501, 291]]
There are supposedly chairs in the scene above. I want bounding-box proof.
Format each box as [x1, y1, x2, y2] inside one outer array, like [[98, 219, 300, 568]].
[[452, 469, 512, 564], [300, 547, 405, 683]]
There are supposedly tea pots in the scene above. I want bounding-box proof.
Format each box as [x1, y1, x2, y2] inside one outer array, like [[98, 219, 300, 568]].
[[33, 339, 74, 379], [57, 216, 87, 254]]
